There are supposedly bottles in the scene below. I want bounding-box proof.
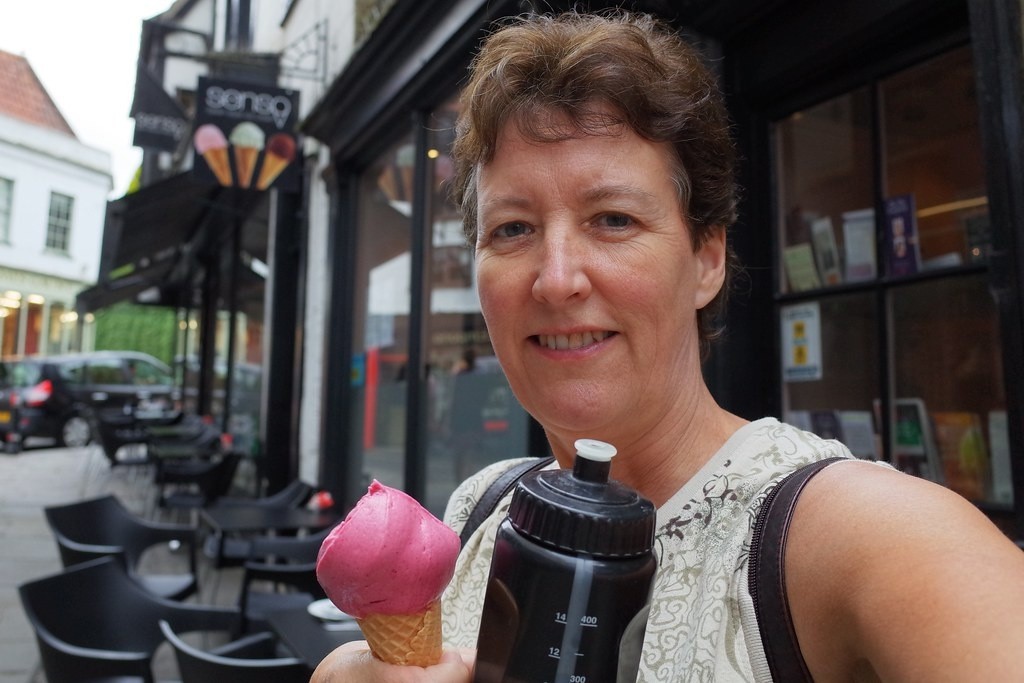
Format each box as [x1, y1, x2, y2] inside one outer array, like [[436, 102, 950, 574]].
[[473, 438, 661, 683]]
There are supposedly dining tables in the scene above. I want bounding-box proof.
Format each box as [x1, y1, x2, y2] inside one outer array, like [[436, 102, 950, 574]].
[[249, 589, 369, 675], [201, 504, 337, 539]]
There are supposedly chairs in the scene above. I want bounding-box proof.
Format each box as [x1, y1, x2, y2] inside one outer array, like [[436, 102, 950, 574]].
[[213, 481, 311, 507], [46, 489, 200, 606], [158, 619, 310, 683], [236, 522, 340, 608], [150, 451, 244, 526], [16, 556, 245, 683]]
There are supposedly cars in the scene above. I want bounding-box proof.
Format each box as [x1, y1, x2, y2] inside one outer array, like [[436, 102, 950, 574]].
[[0, 350, 175, 456]]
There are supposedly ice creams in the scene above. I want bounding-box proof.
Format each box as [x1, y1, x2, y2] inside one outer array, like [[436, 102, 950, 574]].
[[315, 478, 461, 666], [192, 122, 296, 192]]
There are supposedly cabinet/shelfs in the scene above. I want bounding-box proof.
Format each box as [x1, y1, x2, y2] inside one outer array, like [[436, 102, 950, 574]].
[[784, 256, 1024, 544]]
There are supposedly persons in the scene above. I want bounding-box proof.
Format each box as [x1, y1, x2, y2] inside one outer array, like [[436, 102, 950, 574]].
[[308, 7, 1024, 682]]
